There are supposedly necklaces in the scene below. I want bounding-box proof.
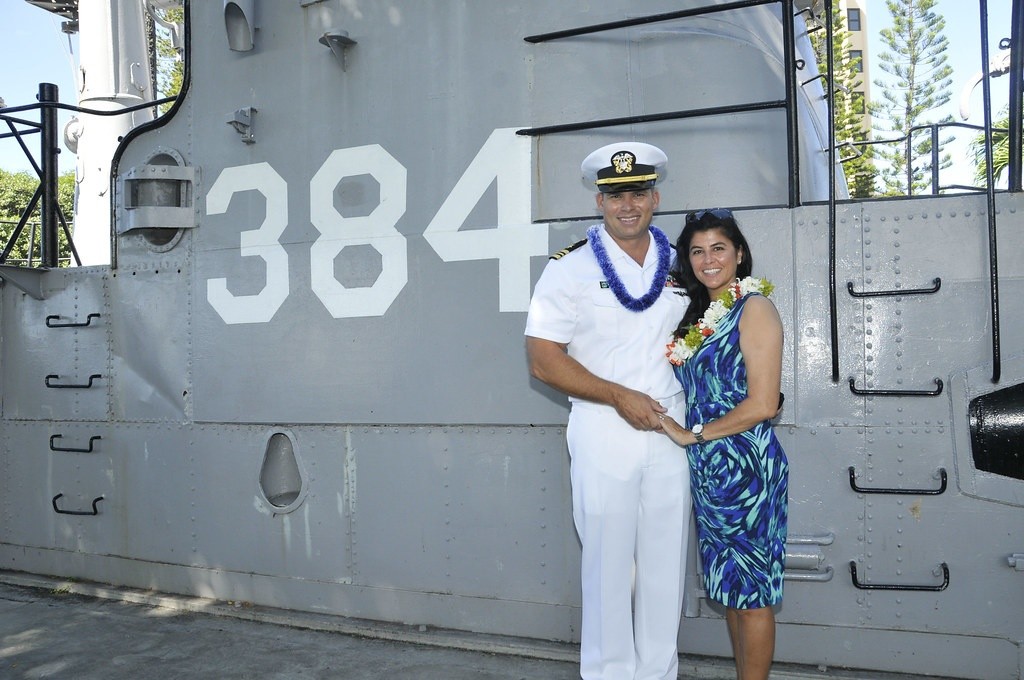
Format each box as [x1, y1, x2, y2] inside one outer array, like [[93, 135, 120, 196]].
[[586, 222, 670, 311], [666, 274, 776, 366]]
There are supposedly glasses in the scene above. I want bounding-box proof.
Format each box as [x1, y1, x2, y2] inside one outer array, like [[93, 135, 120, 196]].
[[686, 209, 733, 223]]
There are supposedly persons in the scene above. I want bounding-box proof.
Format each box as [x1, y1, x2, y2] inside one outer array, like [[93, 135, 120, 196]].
[[652, 210, 789, 680], [523, 141, 695, 680]]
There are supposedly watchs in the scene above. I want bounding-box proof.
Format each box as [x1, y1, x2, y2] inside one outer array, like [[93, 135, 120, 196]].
[[691, 423, 707, 445]]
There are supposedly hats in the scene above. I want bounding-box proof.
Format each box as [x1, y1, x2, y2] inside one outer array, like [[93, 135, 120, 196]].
[[581, 143, 670, 194]]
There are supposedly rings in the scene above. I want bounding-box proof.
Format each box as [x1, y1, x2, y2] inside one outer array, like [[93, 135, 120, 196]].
[[658, 415, 664, 420]]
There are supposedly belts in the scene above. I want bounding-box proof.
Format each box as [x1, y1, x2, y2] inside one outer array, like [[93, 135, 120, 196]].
[[658, 392, 685, 409]]
